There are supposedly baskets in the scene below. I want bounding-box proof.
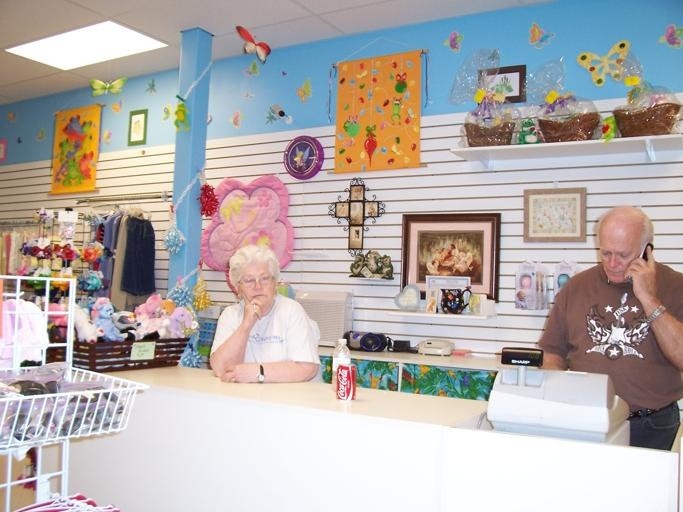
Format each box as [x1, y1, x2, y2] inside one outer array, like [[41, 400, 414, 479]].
[[536, 112, 602, 144], [463, 120, 516, 147], [0, 366, 149, 449], [49, 335, 191, 374], [613, 102, 681, 138]]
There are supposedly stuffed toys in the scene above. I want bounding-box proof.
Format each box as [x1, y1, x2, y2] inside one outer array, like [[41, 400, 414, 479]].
[[3, 374, 123, 442], [14, 238, 111, 293], [48, 293, 192, 342]]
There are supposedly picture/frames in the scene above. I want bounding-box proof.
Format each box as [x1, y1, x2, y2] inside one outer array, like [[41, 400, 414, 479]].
[[398, 211, 500, 299], [0, 137, 7, 162], [476, 64, 526, 105], [522, 187, 586, 243], [126, 107, 148, 147]]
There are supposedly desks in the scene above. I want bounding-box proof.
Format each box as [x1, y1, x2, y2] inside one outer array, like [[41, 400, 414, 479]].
[[318, 345, 535, 392]]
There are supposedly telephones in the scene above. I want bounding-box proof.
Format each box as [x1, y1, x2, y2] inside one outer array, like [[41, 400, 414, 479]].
[[417, 340, 455, 355]]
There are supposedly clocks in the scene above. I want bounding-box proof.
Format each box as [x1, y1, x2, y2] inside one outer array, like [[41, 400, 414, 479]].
[[282, 134, 324, 181]]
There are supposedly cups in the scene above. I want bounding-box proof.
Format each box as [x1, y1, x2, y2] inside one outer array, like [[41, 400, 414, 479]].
[[469, 293, 487, 316]]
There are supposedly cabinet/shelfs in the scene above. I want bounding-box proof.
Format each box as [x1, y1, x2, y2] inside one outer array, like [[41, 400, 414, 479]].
[[0, 274, 152, 512]]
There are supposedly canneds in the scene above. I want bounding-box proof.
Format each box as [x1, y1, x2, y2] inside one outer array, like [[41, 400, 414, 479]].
[[337, 363, 357, 401]]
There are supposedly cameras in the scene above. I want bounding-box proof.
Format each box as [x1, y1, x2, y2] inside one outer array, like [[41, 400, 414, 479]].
[[343, 331, 387, 352]]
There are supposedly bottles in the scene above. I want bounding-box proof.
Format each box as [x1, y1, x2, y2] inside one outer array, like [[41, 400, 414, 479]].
[[332, 337, 352, 392]]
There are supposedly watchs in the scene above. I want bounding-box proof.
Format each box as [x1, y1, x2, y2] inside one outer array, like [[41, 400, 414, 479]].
[[257, 365, 265, 382]]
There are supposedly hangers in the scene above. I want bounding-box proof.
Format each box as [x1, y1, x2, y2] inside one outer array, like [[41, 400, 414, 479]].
[[87, 198, 150, 223]]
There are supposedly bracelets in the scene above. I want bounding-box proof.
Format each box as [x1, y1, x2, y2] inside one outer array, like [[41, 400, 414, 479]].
[[647, 304, 666, 323]]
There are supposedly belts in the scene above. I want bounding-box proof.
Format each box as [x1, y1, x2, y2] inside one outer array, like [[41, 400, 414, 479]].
[[626, 401, 673, 419]]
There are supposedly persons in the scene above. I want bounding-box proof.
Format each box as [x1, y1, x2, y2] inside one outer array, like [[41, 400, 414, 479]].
[[209, 243, 324, 384], [537, 205, 683, 451]]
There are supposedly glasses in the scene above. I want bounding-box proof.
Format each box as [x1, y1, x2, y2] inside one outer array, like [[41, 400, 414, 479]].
[[239, 275, 274, 286]]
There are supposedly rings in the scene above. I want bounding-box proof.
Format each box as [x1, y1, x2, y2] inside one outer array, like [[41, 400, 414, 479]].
[[253, 304, 255, 310]]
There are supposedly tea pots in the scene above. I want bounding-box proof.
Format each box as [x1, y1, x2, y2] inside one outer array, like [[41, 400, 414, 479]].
[[439, 287, 472, 315]]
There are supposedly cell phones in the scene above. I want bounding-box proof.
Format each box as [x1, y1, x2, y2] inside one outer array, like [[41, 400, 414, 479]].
[[639, 242, 654, 262]]
